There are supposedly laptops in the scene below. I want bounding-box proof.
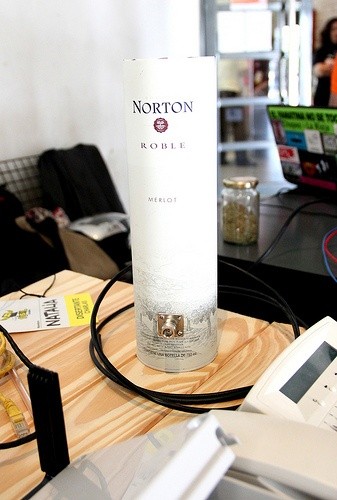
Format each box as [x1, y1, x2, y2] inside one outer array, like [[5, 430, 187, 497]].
[[266, 105, 337, 204]]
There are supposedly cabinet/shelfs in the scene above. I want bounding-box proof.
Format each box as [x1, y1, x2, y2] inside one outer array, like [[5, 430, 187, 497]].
[[200, 1, 284, 176]]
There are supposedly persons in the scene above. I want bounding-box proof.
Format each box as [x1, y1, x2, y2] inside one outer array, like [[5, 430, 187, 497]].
[[312, 16, 337, 109]]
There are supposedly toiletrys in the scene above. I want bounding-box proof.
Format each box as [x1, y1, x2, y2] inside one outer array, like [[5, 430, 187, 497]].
[[124, 55, 218, 375]]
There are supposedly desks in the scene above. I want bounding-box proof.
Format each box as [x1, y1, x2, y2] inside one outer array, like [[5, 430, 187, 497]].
[[0, 269, 308, 500], [218, 179, 337, 277]]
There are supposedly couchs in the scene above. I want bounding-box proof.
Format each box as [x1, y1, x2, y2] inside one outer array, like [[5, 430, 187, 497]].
[[0, 154, 41, 278]]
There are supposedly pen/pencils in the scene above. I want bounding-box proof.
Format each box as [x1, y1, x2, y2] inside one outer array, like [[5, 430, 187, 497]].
[[8, 363, 33, 414]]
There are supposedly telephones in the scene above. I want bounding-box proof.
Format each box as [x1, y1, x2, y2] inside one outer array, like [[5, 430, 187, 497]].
[[201, 315, 337, 500]]
